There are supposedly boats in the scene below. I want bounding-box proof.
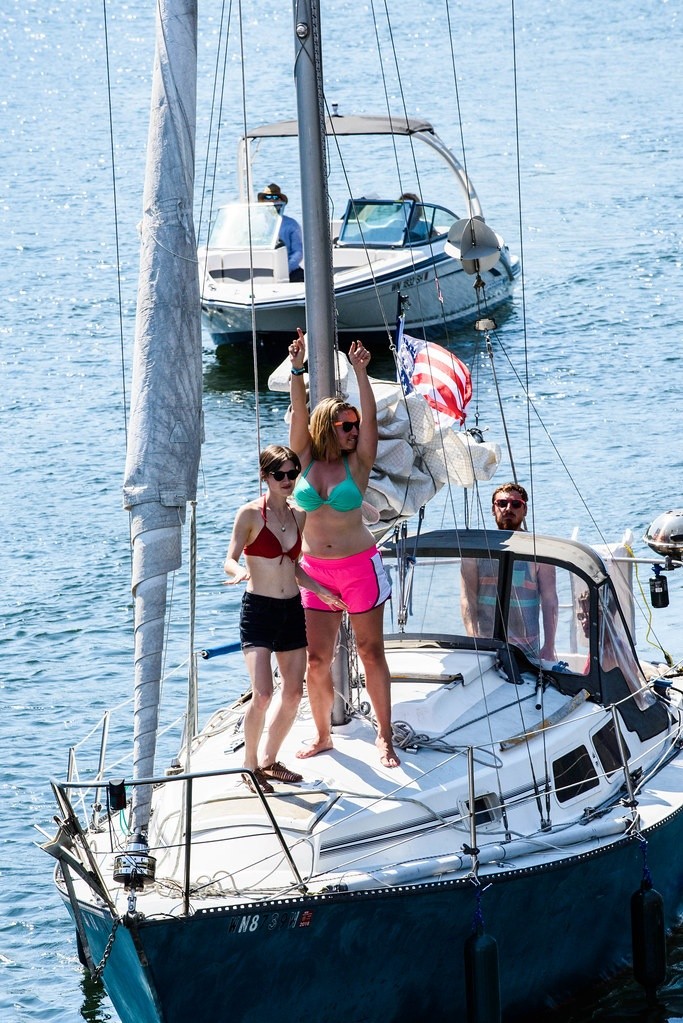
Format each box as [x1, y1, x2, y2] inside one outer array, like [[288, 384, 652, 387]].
[[202, 103, 523, 349], [32, 1, 683, 1023]]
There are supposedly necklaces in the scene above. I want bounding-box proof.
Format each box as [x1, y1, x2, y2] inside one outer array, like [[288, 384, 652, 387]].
[[266, 503, 286, 532]]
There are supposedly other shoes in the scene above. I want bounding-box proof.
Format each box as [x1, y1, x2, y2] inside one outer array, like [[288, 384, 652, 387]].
[[240, 765, 274, 793], [261, 761, 302, 782]]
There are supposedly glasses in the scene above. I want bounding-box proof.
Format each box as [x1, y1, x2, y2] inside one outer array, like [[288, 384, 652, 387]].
[[269, 469, 301, 481], [334, 420, 360, 432], [493, 498, 526, 508], [262, 195, 279, 202], [577, 610, 589, 620]]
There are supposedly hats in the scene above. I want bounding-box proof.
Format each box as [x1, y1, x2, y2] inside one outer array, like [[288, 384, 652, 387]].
[[399, 193, 422, 217], [257, 184, 288, 204]]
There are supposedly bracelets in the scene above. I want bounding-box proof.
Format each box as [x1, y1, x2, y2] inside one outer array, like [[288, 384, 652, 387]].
[[291, 364, 306, 375]]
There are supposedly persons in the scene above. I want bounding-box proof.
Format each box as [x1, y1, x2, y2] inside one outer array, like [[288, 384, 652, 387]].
[[257, 184, 304, 282], [388, 193, 437, 241], [460, 483, 560, 663], [287, 326, 401, 770], [221, 442, 350, 793]]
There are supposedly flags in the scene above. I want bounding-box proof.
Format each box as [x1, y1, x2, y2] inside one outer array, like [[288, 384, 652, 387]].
[[397, 313, 472, 425]]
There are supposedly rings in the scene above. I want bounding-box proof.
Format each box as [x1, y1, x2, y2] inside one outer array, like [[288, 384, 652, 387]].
[[335, 601, 339, 605]]
